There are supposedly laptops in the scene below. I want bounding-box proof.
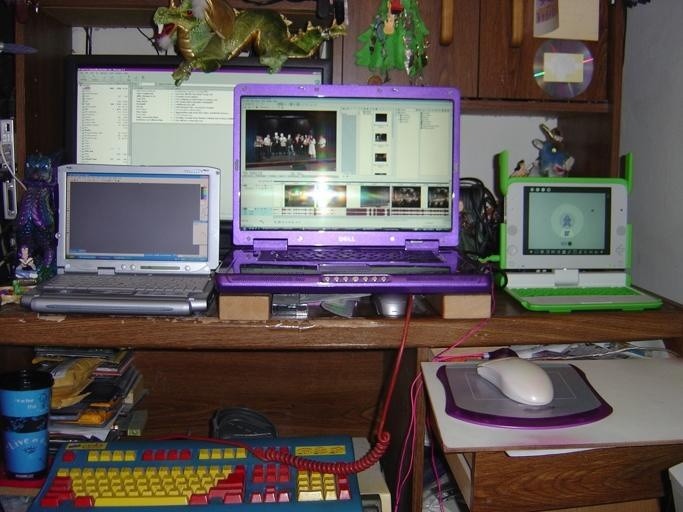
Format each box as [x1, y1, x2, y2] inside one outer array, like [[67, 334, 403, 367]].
[[19, 164, 221, 317], [502, 175, 662, 315], [213, 83, 494, 295]]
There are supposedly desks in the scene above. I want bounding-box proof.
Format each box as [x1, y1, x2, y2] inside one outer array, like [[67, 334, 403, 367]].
[[0, 284, 682, 512]]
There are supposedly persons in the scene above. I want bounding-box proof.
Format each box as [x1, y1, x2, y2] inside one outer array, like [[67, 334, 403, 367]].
[[252, 130, 326, 162]]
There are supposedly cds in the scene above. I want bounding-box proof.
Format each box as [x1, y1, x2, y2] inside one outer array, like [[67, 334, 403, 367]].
[[532, 37, 594, 99]]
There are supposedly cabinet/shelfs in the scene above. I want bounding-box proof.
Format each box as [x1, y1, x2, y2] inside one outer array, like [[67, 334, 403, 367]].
[[0, 1, 72, 285], [330, 0, 625, 112], [31, 0, 317, 30]]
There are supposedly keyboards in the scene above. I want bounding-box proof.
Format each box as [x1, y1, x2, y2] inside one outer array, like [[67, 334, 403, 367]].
[[31, 436, 364, 511]]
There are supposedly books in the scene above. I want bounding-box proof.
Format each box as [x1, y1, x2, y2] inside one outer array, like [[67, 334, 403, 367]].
[[29, 343, 150, 456]]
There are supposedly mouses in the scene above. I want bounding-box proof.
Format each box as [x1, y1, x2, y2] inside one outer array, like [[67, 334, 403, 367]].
[[478, 360, 555, 407], [375, 297, 415, 319]]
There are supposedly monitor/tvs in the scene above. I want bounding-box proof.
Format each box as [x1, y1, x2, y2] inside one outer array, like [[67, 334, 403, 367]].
[[64, 54, 332, 237]]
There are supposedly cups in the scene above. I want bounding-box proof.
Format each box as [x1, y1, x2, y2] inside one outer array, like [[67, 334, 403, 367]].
[[0, 367, 54, 482]]
